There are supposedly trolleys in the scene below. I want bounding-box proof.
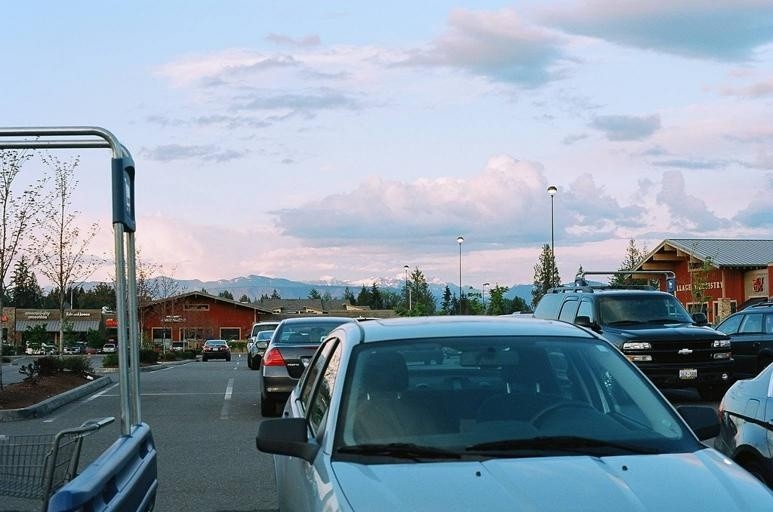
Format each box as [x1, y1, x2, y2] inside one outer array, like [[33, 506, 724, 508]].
[[0, 416, 115, 512]]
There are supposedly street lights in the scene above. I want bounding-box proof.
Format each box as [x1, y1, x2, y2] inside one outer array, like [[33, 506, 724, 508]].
[[402, 264, 409, 305], [454, 234, 465, 301], [546, 184, 557, 290]]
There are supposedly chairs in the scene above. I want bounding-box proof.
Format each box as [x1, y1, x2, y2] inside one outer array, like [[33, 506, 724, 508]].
[[354, 349, 438, 439], [477, 343, 569, 424]]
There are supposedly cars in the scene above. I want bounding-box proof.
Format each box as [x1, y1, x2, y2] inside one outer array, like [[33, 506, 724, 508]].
[[200, 339, 231, 363], [23, 340, 116, 355]]
